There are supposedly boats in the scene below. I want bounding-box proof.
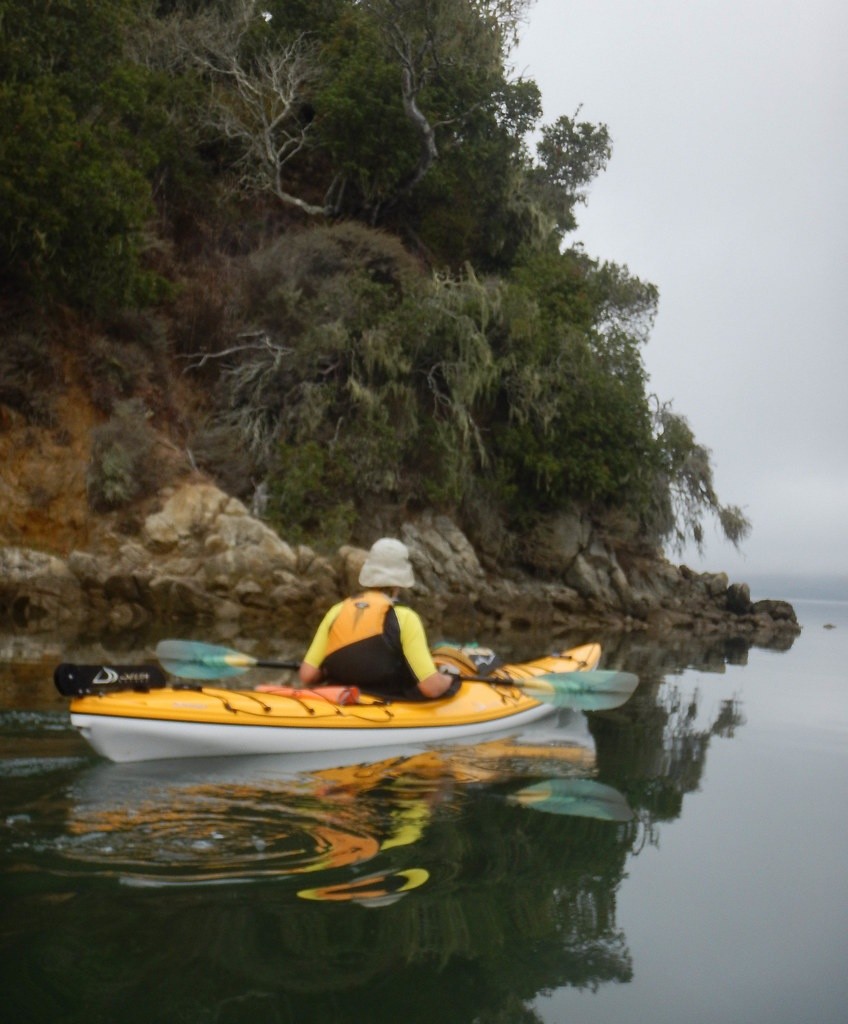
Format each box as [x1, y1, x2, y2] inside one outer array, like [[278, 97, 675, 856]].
[[55, 641, 604, 762]]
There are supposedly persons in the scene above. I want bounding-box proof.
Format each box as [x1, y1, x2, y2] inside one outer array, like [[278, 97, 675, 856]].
[[299, 537, 460, 705]]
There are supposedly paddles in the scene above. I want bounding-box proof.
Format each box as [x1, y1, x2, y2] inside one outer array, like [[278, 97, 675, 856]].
[[466, 779, 636, 822], [156, 638, 639, 711]]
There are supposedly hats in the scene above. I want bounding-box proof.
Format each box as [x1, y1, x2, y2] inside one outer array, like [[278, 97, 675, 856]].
[[359, 537, 414, 589]]
